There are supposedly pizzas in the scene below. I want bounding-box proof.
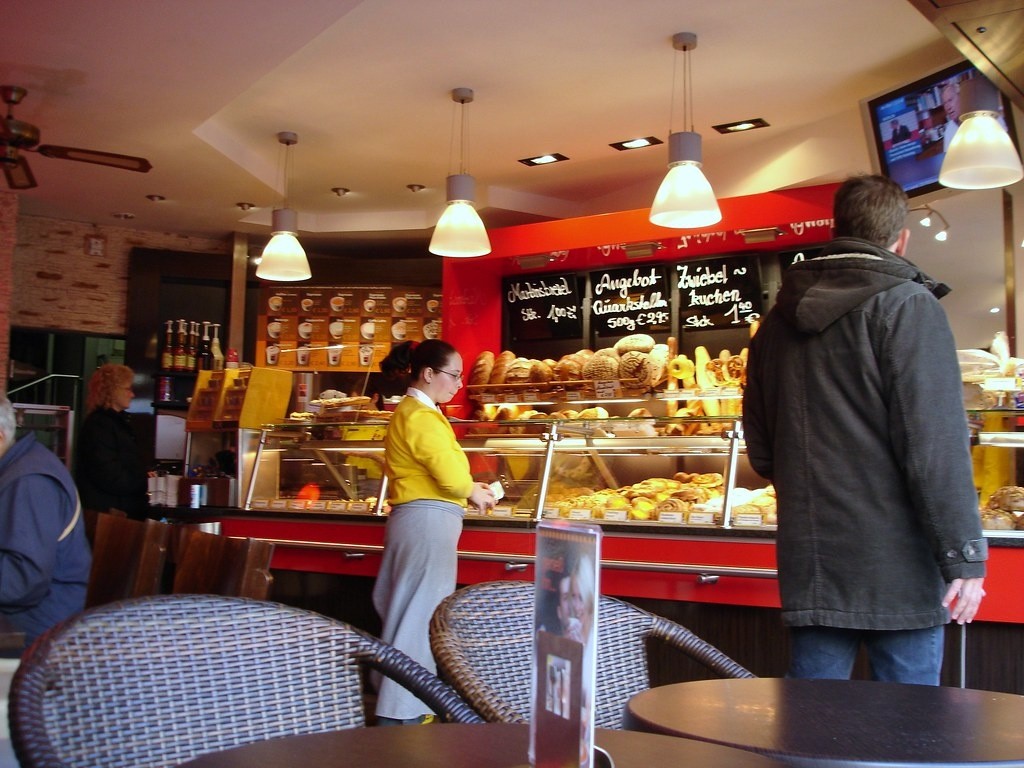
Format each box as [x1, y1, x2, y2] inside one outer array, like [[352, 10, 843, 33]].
[[289, 395, 394, 419]]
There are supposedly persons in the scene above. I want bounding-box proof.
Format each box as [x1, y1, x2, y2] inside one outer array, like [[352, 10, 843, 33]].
[[377, 340, 421, 398], [743, 175, 989, 686], [0, 390, 90, 658], [78, 363, 150, 521], [373, 339, 495, 726], [889, 83, 1008, 155]]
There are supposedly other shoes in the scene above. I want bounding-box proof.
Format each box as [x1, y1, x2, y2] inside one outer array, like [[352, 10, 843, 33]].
[[377, 714, 434, 727]]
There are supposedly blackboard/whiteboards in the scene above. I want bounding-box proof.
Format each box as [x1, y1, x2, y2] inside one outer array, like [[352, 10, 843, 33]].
[[500, 272, 583, 341], [588, 264, 674, 337], [677, 254, 765, 332]]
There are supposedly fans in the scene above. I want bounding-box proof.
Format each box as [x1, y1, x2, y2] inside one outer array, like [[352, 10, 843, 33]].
[[0, 84, 153, 189]]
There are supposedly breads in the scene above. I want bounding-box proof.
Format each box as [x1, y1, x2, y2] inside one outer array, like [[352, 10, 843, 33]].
[[977, 485, 1024, 531], [471, 334, 783, 524]]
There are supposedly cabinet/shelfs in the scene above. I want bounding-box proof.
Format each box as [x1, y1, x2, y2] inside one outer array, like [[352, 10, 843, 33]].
[[186, 367, 294, 432]]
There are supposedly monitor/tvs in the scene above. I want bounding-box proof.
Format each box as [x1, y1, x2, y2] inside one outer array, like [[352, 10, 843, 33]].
[[858, 54, 1022, 210]]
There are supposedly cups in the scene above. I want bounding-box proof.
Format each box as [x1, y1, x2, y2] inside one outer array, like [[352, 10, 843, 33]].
[[361, 323, 375, 340], [297, 346, 309, 364], [269, 297, 283, 311], [301, 300, 313, 312], [427, 300, 439, 313], [391, 325, 404, 340], [266, 346, 279, 365], [359, 347, 373, 367], [423, 323, 440, 340], [330, 297, 344, 312], [392, 297, 406, 314], [327, 322, 342, 339], [363, 299, 375, 312], [297, 322, 312, 339], [327, 347, 341, 365], [266, 322, 281, 339]]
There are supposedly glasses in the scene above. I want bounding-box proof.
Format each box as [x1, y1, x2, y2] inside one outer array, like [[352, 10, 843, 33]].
[[432, 366, 464, 382], [119, 387, 133, 392]]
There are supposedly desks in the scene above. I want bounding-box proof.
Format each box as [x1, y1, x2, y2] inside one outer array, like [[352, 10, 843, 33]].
[[625, 678, 1024, 768], [180, 723, 767, 768]]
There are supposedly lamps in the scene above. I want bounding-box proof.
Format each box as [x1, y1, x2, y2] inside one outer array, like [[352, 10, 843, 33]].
[[255, 131, 312, 282], [429, 88, 492, 259], [938, 80, 1024, 191], [648, 31, 722, 228]]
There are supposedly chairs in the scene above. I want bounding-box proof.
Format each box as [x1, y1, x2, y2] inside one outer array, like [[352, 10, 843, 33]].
[[431, 581, 759, 722], [89, 512, 171, 594], [7, 594, 474, 768], [172, 530, 272, 601]]
